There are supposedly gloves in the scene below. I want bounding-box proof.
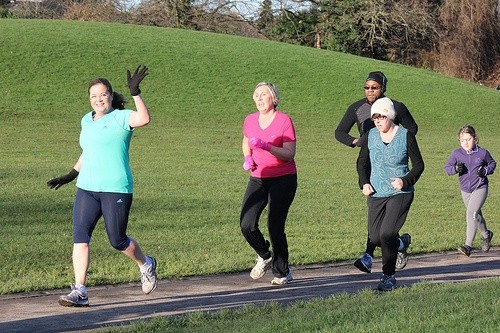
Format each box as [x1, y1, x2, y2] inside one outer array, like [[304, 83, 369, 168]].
[[47, 169, 79, 190], [477, 166, 486, 177], [127, 65, 149, 95], [248, 137, 272, 152], [243, 155, 254, 171], [455, 161, 464, 173]]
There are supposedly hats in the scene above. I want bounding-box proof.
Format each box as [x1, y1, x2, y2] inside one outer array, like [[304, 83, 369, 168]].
[[367, 71, 387, 93], [371, 96, 396, 121]]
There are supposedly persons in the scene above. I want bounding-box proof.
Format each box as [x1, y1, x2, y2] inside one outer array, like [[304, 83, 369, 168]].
[[47, 65, 157, 307], [444, 125, 497, 256], [356, 98, 424, 290], [239, 82, 298, 284], [334, 71, 418, 273]]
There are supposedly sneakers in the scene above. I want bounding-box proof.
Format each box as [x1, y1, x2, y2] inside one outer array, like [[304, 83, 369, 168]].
[[395, 233, 411, 269], [480, 230, 493, 252], [140, 255, 158, 295], [377, 275, 397, 291], [354, 253, 373, 273], [57, 283, 89, 307], [458, 245, 472, 257], [249, 251, 273, 280], [271, 271, 293, 285]]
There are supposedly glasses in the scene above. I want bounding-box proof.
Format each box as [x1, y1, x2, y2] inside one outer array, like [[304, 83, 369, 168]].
[[372, 116, 386, 120], [364, 87, 378, 89]]
